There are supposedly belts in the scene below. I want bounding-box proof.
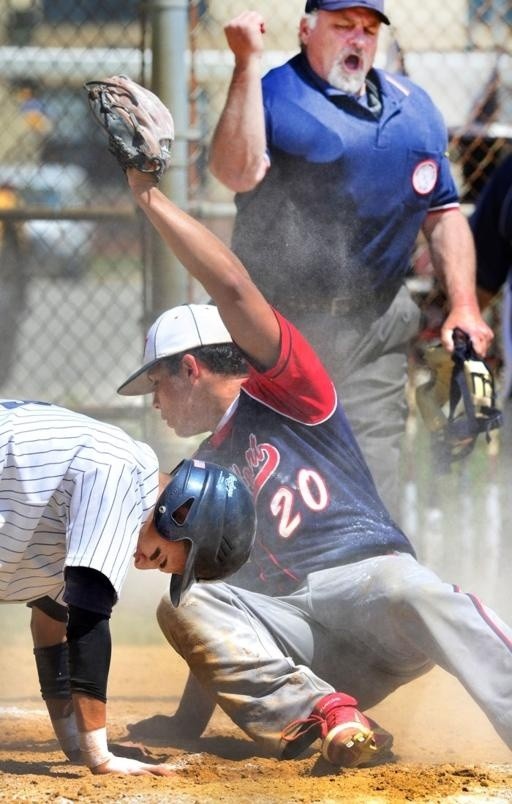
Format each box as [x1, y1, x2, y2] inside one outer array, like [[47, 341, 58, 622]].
[[277, 290, 372, 318]]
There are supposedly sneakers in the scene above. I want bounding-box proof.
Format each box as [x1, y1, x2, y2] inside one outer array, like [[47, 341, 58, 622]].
[[280, 690, 394, 769]]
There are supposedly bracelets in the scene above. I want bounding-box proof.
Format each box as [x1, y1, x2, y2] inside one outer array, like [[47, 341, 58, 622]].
[[49, 713, 84, 763], [81, 726, 115, 773]]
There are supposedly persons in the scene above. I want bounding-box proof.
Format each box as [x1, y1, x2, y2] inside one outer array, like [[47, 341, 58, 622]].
[[1, 391, 261, 780], [201, 0, 498, 537], [81, 69, 512, 770], [420, 136, 511, 336]]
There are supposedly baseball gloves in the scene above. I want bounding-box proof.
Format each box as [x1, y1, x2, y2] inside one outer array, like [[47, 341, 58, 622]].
[[85, 73, 174, 185]]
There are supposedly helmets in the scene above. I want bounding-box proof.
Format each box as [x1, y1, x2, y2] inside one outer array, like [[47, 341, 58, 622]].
[[151, 456, 258, 609]]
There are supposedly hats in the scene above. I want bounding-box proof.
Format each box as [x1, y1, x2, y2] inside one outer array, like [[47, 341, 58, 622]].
[[116, 302, 235, 396], [304, 0, 391, 26]]
